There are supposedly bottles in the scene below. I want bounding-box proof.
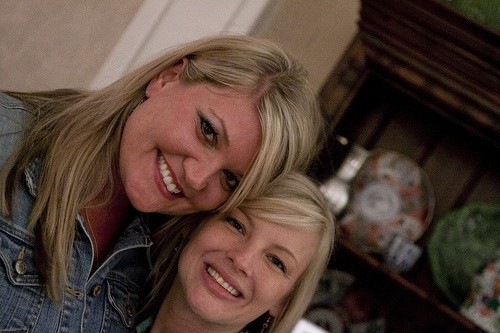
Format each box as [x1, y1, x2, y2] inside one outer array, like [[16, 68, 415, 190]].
[[318, 146, 373, 216]]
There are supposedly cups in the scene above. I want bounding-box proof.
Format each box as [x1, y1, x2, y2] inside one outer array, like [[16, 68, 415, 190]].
[[377, 228, 421, 273]]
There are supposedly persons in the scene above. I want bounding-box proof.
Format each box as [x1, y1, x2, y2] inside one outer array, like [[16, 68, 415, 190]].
[[144, 170, 335, 332], [3, 31, 322, 333]]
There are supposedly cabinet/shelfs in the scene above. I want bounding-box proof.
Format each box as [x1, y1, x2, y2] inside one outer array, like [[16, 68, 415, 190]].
[[296, 0, 500, 333]]
